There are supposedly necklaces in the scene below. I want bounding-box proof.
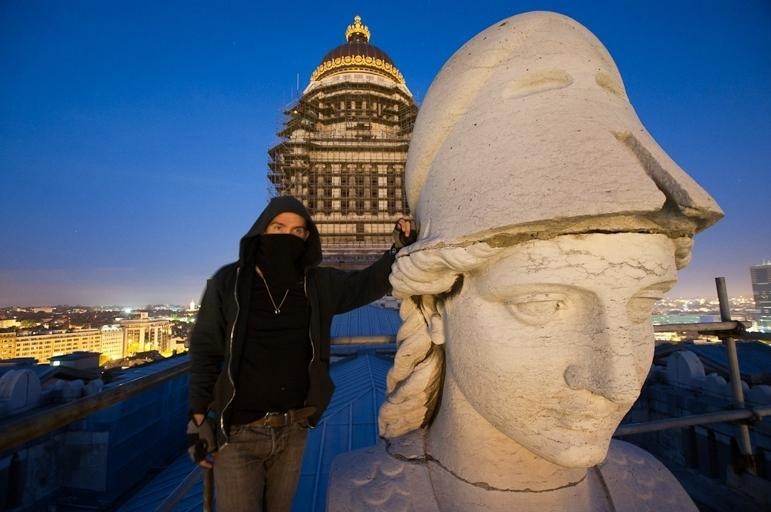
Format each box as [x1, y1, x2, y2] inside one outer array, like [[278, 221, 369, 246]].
[[255, 264, 289, 315]]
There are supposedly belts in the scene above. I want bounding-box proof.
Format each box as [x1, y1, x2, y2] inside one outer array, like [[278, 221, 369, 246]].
[[245, 406, 318, 428]]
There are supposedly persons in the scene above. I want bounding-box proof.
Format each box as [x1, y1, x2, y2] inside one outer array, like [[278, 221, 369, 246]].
[[321, 10, 728, 511], [183, 194, 423, 512]]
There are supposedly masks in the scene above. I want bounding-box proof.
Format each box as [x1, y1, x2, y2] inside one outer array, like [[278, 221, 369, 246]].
[[259, 234, 308, 269]]
